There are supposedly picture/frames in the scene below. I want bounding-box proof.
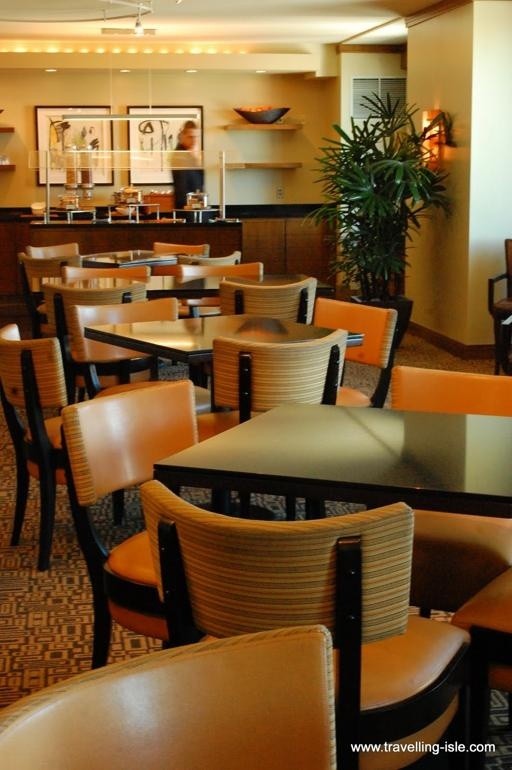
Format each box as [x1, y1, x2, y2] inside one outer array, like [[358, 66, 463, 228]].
[[126, 105, 204, 185], [34, 106, 114, 186]]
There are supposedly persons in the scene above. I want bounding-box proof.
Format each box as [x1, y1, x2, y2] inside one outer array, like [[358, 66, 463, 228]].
[[171, 120, 204, 209]]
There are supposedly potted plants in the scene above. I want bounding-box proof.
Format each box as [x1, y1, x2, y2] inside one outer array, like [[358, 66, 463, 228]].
[[300, 90, 457, 348]]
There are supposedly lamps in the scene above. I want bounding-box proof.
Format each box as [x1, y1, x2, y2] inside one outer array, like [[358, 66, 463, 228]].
[[422, 105, 449, 164], [134, 1, 148, 34]]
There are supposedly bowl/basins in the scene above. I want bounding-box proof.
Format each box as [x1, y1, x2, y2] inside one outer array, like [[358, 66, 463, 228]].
[[231, 107, 290, 124]]
